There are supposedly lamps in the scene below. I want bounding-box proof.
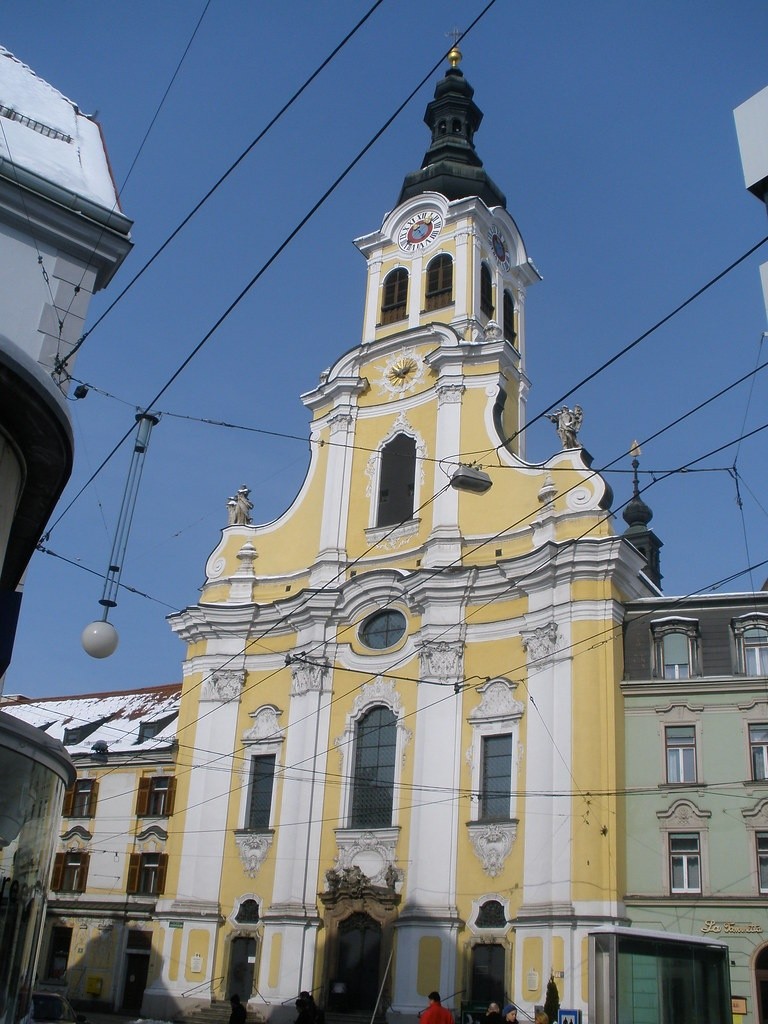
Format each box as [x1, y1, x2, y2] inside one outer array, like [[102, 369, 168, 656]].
[[451, 465, 493, 494], [79, 599, 123, 657], [91, 742, 109, 754]]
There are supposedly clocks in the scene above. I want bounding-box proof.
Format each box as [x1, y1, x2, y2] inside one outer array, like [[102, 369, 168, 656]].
[[398, 210, 443, 252], [486, 223, 512, 272]]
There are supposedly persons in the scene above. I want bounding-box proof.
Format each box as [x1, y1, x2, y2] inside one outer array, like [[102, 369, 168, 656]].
[[228, 993, 248, 1024], [418, 992, 455, 1024], [535, 1011, 549, 1024], [295, 991, 325, 1024], [480, 1002, 519, 1024]]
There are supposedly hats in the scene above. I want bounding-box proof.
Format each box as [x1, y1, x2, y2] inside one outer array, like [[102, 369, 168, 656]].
[[428, 991, 440, 1001], [502, 1004, 517, 1017], [230, 994, 241, 1002]]
